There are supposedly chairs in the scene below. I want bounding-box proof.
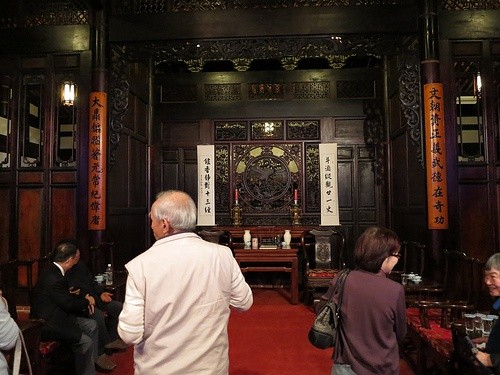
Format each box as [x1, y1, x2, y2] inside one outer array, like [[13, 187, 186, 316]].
[[0, 257, 46, 375], [91, 241, 127, 302], [385, 240, 426, 309], [404, 247, 475, 330], [198, 230, 233, 250], [300, 228, 346, 308], [441, 257, 486, 331]]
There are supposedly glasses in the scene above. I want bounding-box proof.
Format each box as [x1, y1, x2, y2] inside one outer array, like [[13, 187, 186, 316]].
[[391, 254, 401, 259]]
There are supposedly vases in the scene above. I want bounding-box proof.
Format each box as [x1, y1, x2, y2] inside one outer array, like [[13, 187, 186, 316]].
[[243, 229, 251, 250], [283, 229, 292, 250]]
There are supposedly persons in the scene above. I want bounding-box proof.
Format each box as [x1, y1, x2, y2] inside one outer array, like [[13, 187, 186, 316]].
[[117, 190, 253, 375], [471, 254, 500, 375], [0, 289, 20, 375], [316, 227, 407, 375], [27, 243, 131, 375]]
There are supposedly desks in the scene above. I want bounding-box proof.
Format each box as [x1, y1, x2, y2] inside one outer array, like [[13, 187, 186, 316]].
[[233, 244, 299, 305]]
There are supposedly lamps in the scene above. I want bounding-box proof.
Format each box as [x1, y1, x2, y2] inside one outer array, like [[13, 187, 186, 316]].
[[474, 70, 482, 95], [62, 80, 75, 107]]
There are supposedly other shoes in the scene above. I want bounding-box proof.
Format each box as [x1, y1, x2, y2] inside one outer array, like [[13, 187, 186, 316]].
[[106, 339, 129, 348], [95, 354, 116, 369]]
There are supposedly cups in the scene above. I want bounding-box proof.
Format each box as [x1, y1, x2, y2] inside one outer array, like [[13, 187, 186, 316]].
[[94, 271, 111, 284], [464, 313, 499, 336], [408, 272, 418, 280], [401, 274, 408, 284], [412, 274, 423, 284], [252, 238, 259, 249]]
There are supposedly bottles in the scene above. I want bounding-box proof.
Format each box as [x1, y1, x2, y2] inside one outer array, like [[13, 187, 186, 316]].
[[284, 230, 291, 250], [243, 230, 251, 249], [106, 264, 113, 285]]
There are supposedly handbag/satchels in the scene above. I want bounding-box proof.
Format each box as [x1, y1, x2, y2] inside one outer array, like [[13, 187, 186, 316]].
[[307, 268, 352, 350]]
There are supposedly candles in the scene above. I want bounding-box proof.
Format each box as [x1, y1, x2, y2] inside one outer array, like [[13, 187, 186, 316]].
[[294, 189, 298, 200], [235, 189, 239, 200]]
